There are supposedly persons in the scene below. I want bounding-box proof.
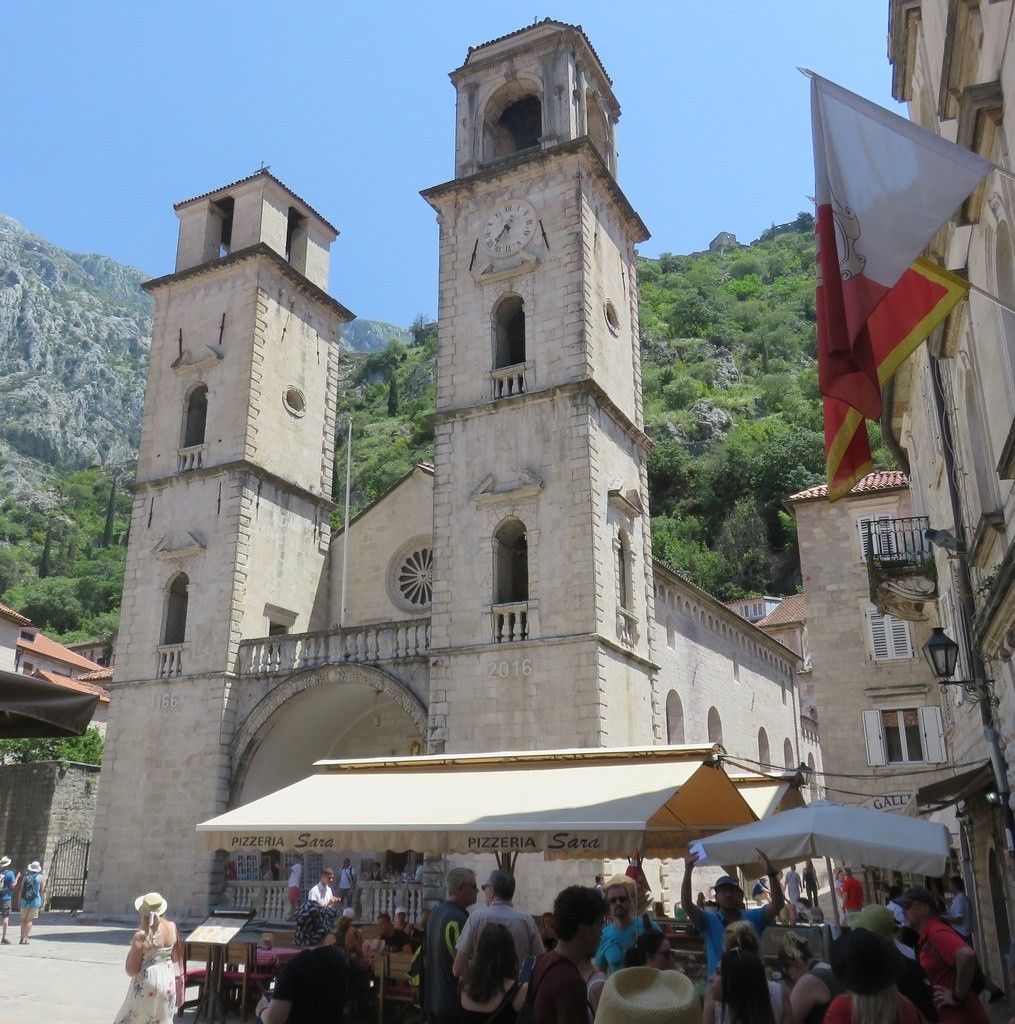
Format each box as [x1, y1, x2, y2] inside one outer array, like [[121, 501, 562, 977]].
[[113, 892, 178, 1024], [18, 861, 45, 945], [255, 848, 989, 1024], [0, 855, 21, 945]]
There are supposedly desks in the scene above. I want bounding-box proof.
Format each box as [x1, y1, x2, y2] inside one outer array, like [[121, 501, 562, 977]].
[[760, 922, 831, 964], [176, 947, 302, 1017]]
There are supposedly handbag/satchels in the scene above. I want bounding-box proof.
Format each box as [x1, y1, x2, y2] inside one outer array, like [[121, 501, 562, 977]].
[[175, 932, 184, 1007]]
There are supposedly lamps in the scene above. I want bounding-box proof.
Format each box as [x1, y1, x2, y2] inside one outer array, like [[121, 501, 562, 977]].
[[921, 627, 999, 707], [986, 792, 1004, 805], [373, 690, 383, 726]]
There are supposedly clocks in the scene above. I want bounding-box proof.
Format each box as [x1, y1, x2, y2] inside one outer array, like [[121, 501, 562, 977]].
[[478, 199, 536, 257]]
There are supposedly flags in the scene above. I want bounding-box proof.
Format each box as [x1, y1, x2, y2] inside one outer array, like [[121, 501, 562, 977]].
[[824, 256, 973, 502], [811, 74, 997, 424]]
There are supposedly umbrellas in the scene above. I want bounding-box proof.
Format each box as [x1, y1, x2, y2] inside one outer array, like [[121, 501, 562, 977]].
[[0, 670, 100, 740], [687, 798, 953, 937]]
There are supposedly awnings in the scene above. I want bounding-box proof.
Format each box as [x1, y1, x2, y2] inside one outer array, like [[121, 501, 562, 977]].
[[545, 770, 807, 861], [900, 762, 995, 819], [197, 744, 760, 853]]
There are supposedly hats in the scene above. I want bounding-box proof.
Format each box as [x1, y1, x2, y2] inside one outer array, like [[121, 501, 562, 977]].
[[293, 900, 338, 946], [830, 928, 906, 995], [715, 876, 739, 892], [593, 966, 704, 1024], [134, 892, 167, 927], [0, 856, 11, 868], [893, 886, 940, 914], [395, 907, 407, 915], [846, 904, 896, 947], [28, 861, 41, 872]]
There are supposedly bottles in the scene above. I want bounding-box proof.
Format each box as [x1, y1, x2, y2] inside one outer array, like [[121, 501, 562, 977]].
[[779, 903, 796, 926]]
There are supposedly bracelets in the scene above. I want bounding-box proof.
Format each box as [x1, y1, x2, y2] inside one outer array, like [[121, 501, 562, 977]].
[[951, 989, 966, 1002], [258, 1007, 269, 1019]]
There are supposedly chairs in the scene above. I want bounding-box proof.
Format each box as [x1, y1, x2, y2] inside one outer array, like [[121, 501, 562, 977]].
[[178, 931, 299, 1024], [362, 923, 415, 1024]]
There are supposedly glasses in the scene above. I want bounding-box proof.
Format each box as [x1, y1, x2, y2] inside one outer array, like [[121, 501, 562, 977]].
[[731, 944, 744, 970], [462, 885, 476, 889], [482, 885, 491, 891], [655, 951, 674, 959], [903, 902, 924, 909], [607, 896, 627, 905]]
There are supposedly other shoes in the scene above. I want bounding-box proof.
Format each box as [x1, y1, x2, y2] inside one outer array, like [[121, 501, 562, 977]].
[[19, 939, 29, 944], [1, 938, 11, 944]]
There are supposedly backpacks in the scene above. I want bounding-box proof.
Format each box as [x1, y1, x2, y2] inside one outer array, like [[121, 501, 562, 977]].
[[22, 873, 42, 904], [0, 870, 10, 896]]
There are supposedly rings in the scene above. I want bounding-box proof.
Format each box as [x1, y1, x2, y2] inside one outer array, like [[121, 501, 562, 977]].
[[940, 995, 943, 1000]]
